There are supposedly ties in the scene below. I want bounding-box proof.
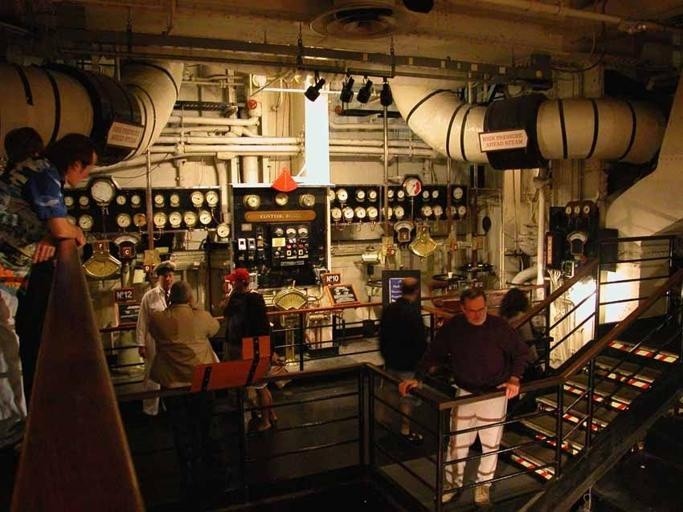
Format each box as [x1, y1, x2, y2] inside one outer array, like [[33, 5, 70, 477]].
[[165, 291, 169, 307]]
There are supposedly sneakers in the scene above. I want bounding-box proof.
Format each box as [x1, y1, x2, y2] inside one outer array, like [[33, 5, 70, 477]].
[[433, 482, 465, 504], [473, 485, 491, 508]]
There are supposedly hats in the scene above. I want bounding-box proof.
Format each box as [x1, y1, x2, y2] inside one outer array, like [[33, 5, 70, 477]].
[[223, 268, 250, 282]]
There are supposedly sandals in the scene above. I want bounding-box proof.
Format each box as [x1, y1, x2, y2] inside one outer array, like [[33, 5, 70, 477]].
[[396, 430, 425, 445]]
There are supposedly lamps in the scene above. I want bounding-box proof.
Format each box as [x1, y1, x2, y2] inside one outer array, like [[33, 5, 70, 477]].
[[301, 70, 395, 108]]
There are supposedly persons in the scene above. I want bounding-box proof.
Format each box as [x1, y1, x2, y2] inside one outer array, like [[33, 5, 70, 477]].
[[3, 126, 45, 178], [498, 287, 544, 412], [134, 260, 176, 437], [147, 279, 228, 495], [397, 289, 528, 509], [220, 268, 278, 432], [376, 277, 428, 440], [0, 133, 99, 456]]
